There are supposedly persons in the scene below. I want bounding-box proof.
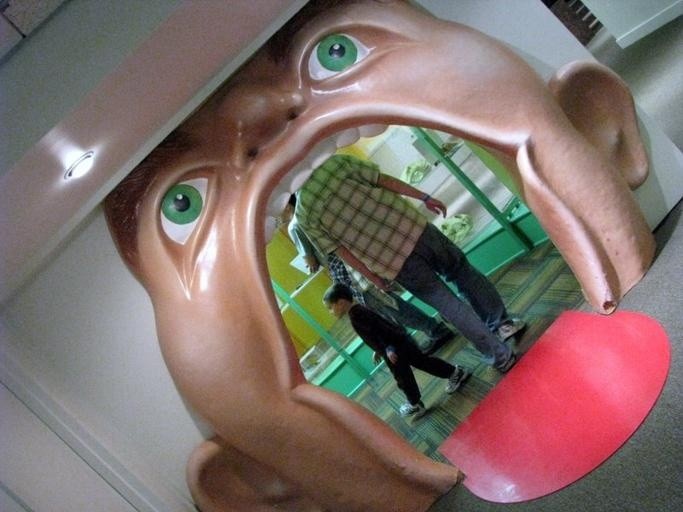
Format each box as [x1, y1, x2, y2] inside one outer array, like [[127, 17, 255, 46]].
[[283, 192, 453, 359], [100, 3, 666, 511], [295, 155, 528, 375], [319, 282, 468, 418]]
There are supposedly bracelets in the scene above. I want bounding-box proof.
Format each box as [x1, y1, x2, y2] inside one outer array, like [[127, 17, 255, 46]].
[[422, 194, 431, 202], [386, 347, 394, 353]]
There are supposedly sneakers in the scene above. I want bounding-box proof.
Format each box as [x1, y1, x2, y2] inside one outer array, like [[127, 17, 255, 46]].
[[400, 319, 528, 418]]
